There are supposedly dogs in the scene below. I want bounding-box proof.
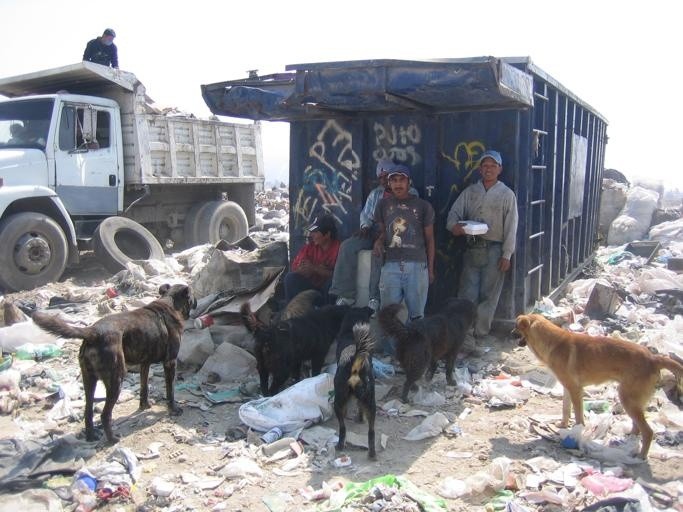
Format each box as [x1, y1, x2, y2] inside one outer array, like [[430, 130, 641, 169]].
[[239, 301, 350, 397], [333, 306, 377, 461], [30, 284, 197, 443], [379, 296, 479, 405], [511, 312, 683, 460]]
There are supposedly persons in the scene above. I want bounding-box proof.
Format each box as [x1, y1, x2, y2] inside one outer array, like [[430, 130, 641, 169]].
[[9, 123, 45, 147], [372, 164, 436, 365], [327, 161, 420, 312], [58, 122, 100, 150], [446, 150, 518, 345], [278, 217, 341, 311], [83, 28, 119, 69]]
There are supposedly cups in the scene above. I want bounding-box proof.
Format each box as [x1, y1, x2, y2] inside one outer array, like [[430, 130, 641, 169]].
[[221, 191, 229, 201], [259, 426, 282, 443]]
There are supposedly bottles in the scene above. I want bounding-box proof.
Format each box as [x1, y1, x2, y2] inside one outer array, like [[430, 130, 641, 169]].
[[583, 401, 609, 411], [193, 313, 213, 330], [15, 343, 57, 361], [333, 457, 351, 468]]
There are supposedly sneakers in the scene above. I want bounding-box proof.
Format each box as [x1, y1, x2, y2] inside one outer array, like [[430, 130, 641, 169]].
[[368, 300, 379, 319], [337, 296, 357, 308]]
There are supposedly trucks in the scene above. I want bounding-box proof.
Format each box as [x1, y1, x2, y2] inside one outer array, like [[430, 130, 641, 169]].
[[0, 62, 262, 296]]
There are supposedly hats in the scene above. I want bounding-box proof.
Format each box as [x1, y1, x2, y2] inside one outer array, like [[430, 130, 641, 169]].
[[479, 151, 502, 166], [376, 159, 397, 177], [389, 166, 410, 178], [303, 215, 336, 232]]
